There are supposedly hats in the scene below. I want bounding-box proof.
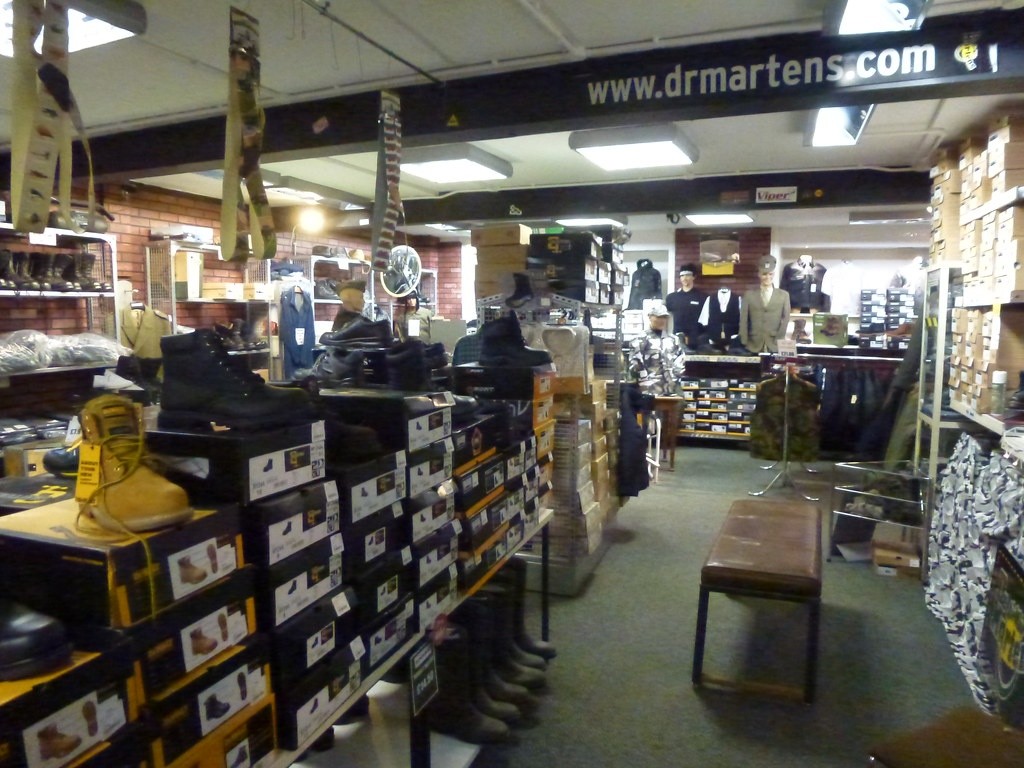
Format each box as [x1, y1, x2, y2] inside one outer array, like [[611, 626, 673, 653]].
[[649, 304, 670, 318], [757, 255, 776, 271], [312, 245, 365, 260], [680, 264, 697, 277]]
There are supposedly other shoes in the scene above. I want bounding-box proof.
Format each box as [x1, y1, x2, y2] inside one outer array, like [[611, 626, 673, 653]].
[[353, 339, 481, 425]]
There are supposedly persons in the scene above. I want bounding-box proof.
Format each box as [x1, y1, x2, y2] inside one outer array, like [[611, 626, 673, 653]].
[[628, 259, 662, 309], [738, 254, 791, 354], [394, 290, 434, 345], [331, 280, 367, 330], [821, 260, 870, 315], [889, 257, 924, 290], [697, 288, 743, 352], [881, 289, 927, 474], [665, 264, 711, 353], [780, 254, 827, 314], [628, 306, 686, 395]]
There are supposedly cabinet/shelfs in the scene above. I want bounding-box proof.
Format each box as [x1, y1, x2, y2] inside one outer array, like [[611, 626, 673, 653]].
[[295, 254, 375, 347], [143, 239, 274, 381], [377, 265, 438, 341], [789, 311, 918, 351], [0, 220, 121, 459], [912, 262, 987, 519], [676, 354, 762, 442], [949, 187, 1024, 437]]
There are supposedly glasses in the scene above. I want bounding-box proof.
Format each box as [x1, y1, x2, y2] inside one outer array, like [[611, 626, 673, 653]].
[[679, 274, 693, 279]]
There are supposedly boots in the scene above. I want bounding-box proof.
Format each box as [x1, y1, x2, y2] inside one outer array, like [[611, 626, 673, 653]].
[[71, 391, 195, 532], [506, 272, 535, 309], [481, 571, 545, 688], [421, 622, 510, 743], [159, 328, 309, 435], [500, 556, 557, 659], [456, 597, 530, 722], [479, 309, 553, 367], [220, 318, 268, 350], [0, 249, 114, 291]]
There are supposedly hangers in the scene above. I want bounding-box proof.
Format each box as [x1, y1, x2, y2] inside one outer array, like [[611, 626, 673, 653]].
[[130, 288, 145, 311], [294, 283, 301, 294]]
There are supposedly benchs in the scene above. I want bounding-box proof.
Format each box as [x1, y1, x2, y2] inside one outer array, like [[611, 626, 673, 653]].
[[865, 708, 1024, 768], [692, 500, 822, 708]]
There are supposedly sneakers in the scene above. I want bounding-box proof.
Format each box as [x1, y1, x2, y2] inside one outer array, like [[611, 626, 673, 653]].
[[41, 446, 80, 480], [320, 318, 395, 350], [292, 352, 366, 387]]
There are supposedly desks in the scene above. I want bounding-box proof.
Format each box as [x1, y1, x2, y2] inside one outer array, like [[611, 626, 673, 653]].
[[270, 509, 555, 768]]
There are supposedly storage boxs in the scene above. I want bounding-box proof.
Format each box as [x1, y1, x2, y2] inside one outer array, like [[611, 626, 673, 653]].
[[887, 323, 899, 331], [929, 113, 1024, 416], [524, 223, 628, 305], [859, 336, 883, 348], [861, 313, 886, 323], [150, 251, 204, 299], [861, 301, 886, 314], [860, 324, 885, 332], [886, 337, 910, 350], [477, 244, 527, 264], [861, 288, 887, 301], [0, 362, 632, 768], [680, 377, 758, 436], [873, 548, 920, 581], [886, 288, 908, 302], [475, 264, 525, 283], [474, 283, 516, 301], [203, 281, 255, 299], [886, 302, 907, 313], [813, 312, 849, 347], [470, 223, 530, 248], [887, 313, 907, 325]]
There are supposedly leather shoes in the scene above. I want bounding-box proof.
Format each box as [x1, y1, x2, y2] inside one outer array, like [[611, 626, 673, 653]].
[[1008, 388, 1024, 409], [1, 585, 77, 682], [885, 324, 913, 337], [856, 323, 885, 336]]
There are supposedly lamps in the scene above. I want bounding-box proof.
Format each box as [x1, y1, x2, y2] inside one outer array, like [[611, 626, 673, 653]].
[[400, 143, 513, 184], [683, 208, 756, 227], [0, 0, 148, 57], [568, 123, 700, 173], [802, 104, 877, 148], [552, 213, 629, 229], [821, 0, 932, 37]]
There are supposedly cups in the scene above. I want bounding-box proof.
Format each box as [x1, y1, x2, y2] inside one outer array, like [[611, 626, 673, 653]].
[[990, 371, 1008, 417]]
